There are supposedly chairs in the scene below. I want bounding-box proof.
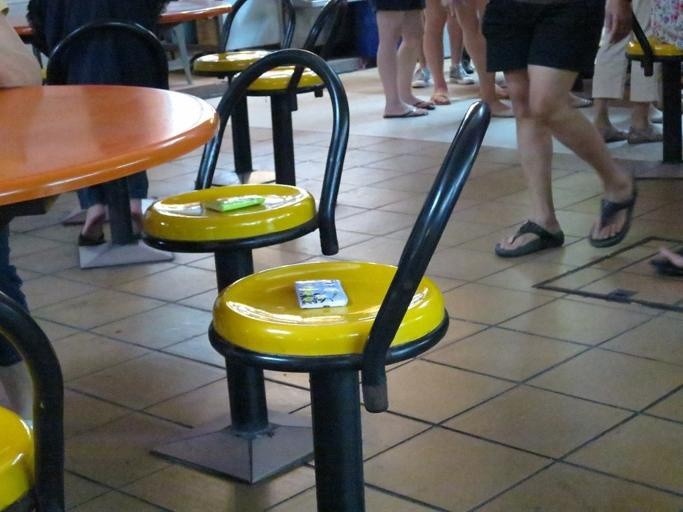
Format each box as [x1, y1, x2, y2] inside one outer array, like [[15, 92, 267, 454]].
[[136, 46, 352, 483], [49, 18, 176, 271], [1, 293, 67, 511], [205, 97, 495, 510], [226, 0, 341, 187], [625, 7, 682, 180], [186, 1, 299, 186]]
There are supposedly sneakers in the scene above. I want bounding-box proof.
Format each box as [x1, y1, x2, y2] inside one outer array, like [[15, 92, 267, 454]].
[[449, 65, 473, 85], [461, 60, 474, 74], [409, 67, 429, 87]]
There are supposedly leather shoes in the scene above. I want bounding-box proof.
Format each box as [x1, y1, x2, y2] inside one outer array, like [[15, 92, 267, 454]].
[[488, 108, 516, 118], [572, 98, 592, 107]]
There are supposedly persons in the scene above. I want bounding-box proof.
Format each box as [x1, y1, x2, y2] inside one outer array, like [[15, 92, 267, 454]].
[[648, 243, 682, 275], [225, 2, 683, 143], [27, 1, 159, 249], [480, 2, 636, 258], [0, 1, 41, 431]]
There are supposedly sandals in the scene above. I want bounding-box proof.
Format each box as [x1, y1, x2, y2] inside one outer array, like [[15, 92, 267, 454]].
[[627, 122, 664, 144], [597, 122, 628, 142]]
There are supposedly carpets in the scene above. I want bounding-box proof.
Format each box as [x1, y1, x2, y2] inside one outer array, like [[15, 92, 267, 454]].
[[345, 97, 665, 161]]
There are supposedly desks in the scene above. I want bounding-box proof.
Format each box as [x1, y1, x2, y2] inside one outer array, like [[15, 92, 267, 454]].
[[1, 84, 217, 512], [12, 3, 233, 84]]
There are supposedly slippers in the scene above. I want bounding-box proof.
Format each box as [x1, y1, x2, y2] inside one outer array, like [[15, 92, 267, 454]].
[[494, 221, 563, 257], [382, 105, 428, 118], [588, 187, 636, 246], [413, 100, 434, 110], [430, 94, 449, 105]]
